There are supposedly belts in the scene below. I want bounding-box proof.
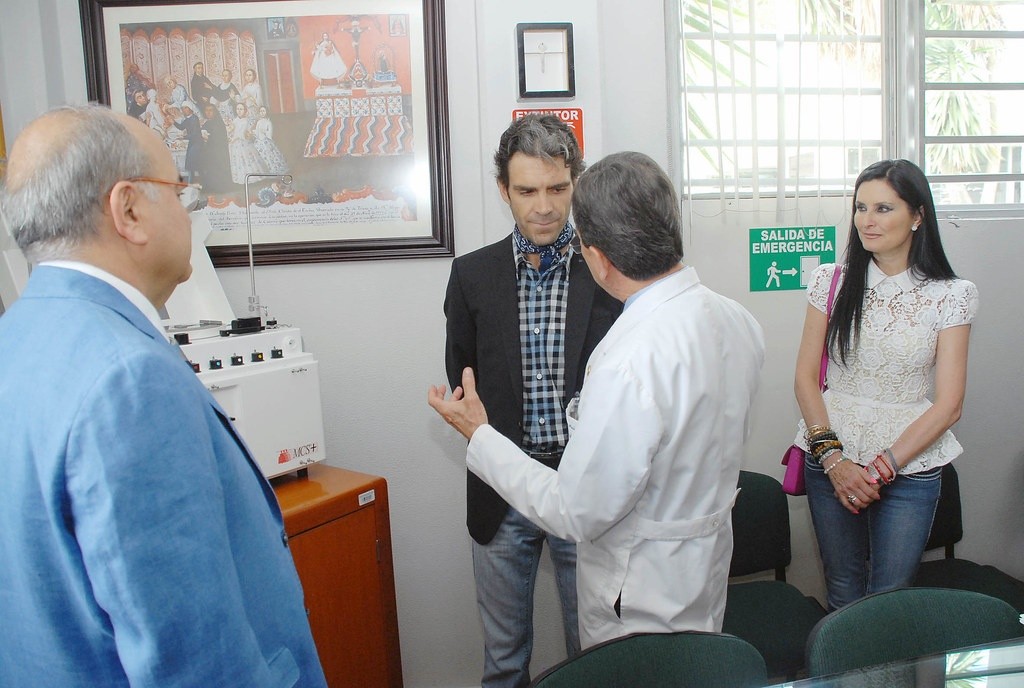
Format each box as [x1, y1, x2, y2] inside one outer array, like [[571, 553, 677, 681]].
[[521, 449, 563, 465]]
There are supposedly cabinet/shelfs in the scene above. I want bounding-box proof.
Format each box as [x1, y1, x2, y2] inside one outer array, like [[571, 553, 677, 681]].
[[269, 463, 403, 688]]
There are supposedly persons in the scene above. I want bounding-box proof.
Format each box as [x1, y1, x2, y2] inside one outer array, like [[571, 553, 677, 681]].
[[0, 100, 332, 688], [442, 115, 625, 688], [427, 152, 767, 652], [792, 158, 978, 615]]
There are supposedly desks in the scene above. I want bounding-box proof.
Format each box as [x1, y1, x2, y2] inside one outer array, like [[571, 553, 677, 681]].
[[767, 635, 1024, 688]]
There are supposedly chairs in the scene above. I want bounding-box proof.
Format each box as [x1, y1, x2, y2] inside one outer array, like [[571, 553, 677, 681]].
[[803, 460, 1024, 609], [805, 587, 1024, 678], [528, 631, 767, 688], [723, 470, 828, 677]]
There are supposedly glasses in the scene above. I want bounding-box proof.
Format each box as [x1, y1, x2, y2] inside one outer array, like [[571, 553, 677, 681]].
[[109, 177, 202, 212]]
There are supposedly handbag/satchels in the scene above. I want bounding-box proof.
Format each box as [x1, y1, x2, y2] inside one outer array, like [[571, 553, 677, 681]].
[[780, 445, 806, 496]]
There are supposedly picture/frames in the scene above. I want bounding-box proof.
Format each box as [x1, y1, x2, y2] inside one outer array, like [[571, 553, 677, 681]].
[[514, 22, 576, 98], [77, 0, 455, 270]]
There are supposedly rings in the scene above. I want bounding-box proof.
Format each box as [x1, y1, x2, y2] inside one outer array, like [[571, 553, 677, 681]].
[[848, 495, 857, 503]]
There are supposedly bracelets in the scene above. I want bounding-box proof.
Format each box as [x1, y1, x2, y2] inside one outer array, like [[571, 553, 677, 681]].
[[864, 448, 899, 491], [803, 425, 843, 465], [823, 456, 846, 475]]
